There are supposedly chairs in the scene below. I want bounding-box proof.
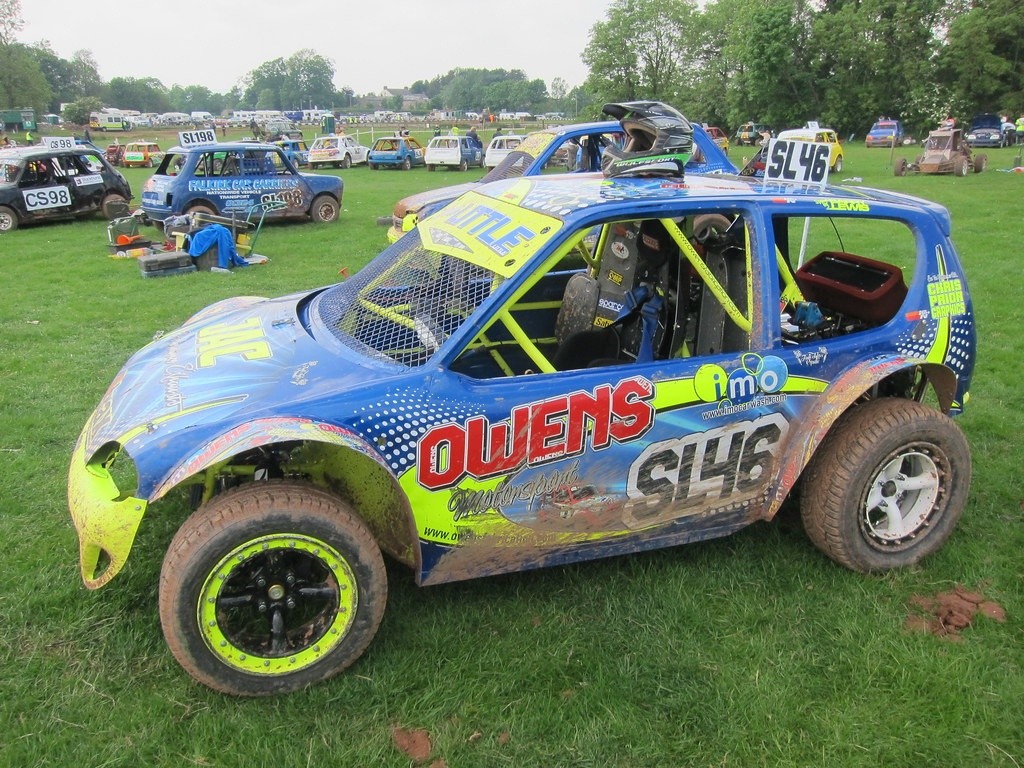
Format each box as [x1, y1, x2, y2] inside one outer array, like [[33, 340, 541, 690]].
[[548, 222, 747, 373]]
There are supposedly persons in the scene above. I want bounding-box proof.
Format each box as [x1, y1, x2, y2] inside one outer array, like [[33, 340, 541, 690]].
[[1001, 122, 1016, 146], [210, 120, 266, 139], [335, 114, 483, 150], [1015, 113, 1024, 144], [493, 128, 504, 149], [759, 129, 770, 147], [26, 129, 33, 146]]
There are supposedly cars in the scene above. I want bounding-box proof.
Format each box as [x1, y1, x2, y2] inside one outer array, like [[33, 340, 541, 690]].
[[69, 179, 978, 698], [0, 120, 1013, 245]]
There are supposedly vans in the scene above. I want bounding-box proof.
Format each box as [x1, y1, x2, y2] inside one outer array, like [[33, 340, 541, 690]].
[[86, 106, 546, 133]]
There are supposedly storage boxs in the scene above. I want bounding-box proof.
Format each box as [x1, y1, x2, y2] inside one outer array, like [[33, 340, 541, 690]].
[[190, 212, 249, 234], [138, 252, 198, 279], [109, 240, 151, 255]]
